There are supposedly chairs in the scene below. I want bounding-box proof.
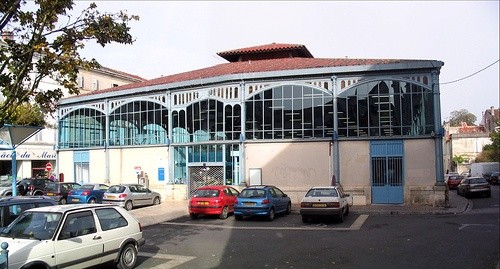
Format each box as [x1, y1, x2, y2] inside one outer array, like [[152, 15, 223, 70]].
[[330, 191, 335, 196], [253, 191, 258, 196], [316, 192, 321, 195]]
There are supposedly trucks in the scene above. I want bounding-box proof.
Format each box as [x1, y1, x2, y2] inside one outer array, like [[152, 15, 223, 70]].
[[467, 161, 500, 178]]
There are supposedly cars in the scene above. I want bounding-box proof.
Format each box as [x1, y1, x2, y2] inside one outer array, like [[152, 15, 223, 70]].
[[102, 183, 161, 211], [0, 203, 145, 269], [42, 181, 81, 204], [444, 172, 468, 190], [188, 185, 240, 220], [0, 194, 59, 233], [233, 185, 292, 222], [66, 183, 110, 204], [481, 171, 500, 185], [0, 178, 22, 197], [299, 186, 350, 224], [456, 177, 491, 199]]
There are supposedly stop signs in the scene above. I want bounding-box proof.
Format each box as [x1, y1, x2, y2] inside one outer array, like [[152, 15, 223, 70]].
[[46, 162, 52, 173]]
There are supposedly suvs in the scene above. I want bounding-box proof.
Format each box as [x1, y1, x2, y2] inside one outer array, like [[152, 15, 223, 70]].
[[16, 177, 55, 196]]
